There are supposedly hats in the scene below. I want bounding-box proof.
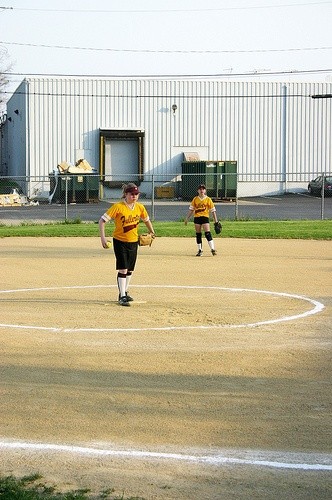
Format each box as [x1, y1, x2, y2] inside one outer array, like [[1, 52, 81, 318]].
[[124, 187, 141, 193], [196, 184, 206, 191]]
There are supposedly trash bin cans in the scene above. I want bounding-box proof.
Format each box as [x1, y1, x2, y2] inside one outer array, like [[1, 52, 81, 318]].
[[49, 171, 72, 204], [86, 173, 99, 202], [66, 172, 85, 203]]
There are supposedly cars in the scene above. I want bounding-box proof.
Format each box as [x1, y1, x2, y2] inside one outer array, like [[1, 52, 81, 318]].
[[0, 181, 27, 205], [308, 175, 332, 196]]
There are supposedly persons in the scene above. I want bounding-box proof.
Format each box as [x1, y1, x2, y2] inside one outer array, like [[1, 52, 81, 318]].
[[99, 183, 156, 306], [185, 185, 220, 256]]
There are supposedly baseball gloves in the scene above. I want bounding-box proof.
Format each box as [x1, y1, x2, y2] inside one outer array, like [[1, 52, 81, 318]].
[[139, 232, 155, 247], [214, 222, 222, 234]]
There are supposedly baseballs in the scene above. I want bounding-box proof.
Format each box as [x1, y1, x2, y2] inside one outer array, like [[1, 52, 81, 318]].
[[107, 242, 111, 247]]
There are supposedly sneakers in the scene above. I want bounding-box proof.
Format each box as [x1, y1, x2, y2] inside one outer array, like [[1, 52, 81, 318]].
[[196, 249, 203, 256], [125, 292, 134, 301], [211, 249, 217, 256], [119, 294, 130, 306]]
